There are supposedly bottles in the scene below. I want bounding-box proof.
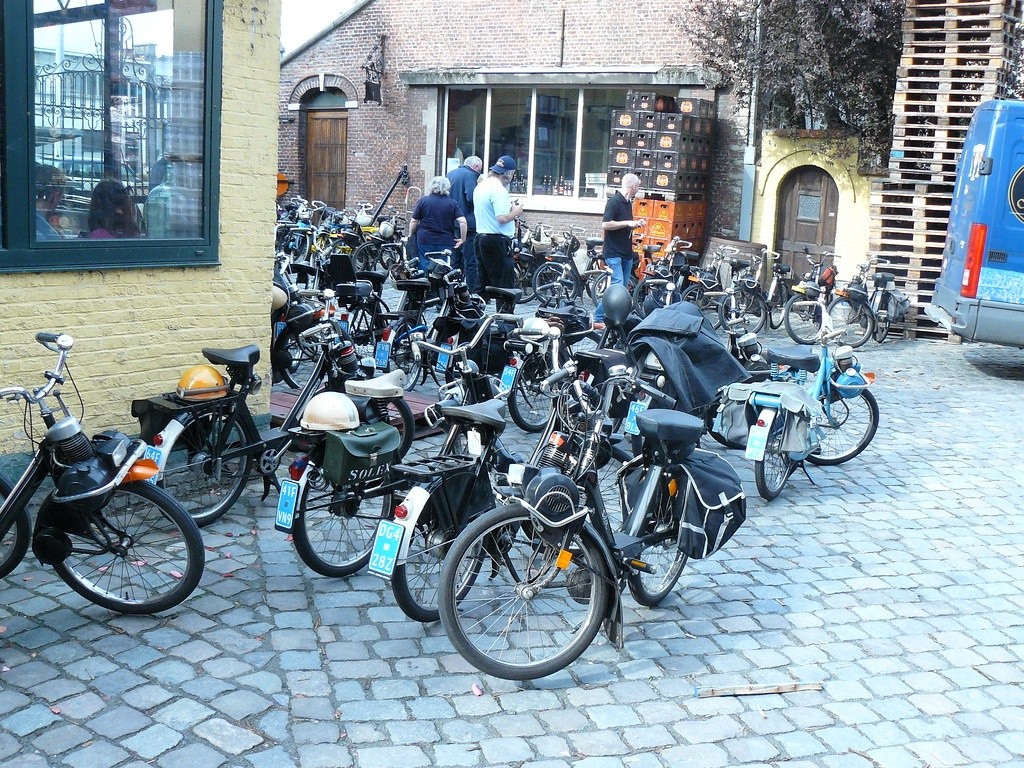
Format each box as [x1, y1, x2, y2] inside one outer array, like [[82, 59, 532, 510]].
[[511, 174, 573, 196]]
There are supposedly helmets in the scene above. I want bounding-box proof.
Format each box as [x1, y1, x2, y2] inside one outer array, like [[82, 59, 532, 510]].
[[519, 317, 550, 340], [176, 365, 227, 401], [354, 215, 373, 226], [379, 221, 394, 238], [300, 391, 359, 430]]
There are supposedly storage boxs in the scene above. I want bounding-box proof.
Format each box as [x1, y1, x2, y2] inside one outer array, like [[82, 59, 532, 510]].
[[603, 92, 716, 263]]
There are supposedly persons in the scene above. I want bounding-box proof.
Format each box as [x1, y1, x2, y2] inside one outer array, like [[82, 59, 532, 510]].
[[473, 155, 525, 313], [593, 173, 646, 323], [82, 179, 139, 240], [408, 155, 482, 296], [37, 164, 65, 240]]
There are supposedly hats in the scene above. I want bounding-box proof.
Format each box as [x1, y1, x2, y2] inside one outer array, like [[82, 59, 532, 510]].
[[489, 155, 516, 174]]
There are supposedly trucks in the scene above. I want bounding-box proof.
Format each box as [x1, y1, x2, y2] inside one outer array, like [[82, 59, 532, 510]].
[[924, 99, 1024, 348]]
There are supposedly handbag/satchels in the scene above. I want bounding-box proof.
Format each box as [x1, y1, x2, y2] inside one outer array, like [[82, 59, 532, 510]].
[[454, 305, 515, 375], [712, 380, 828, 460], [860, 288, 910, 330], [617, 448, 748, 559], [324, 422, 401, 485]]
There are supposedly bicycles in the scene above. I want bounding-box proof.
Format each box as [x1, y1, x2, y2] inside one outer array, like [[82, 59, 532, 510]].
[[272, 195, 910, 679], [131, 289, 416, 529], [0, 332, 205, 613]]
